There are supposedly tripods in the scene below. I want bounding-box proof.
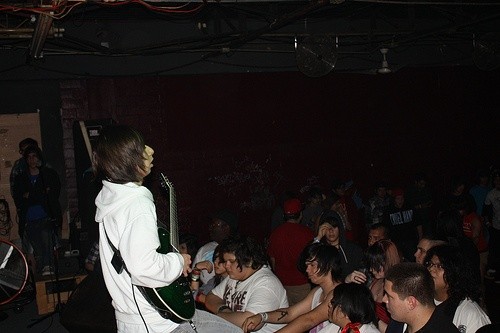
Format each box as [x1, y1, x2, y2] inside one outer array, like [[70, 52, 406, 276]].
[[27, 189, 63, 329]]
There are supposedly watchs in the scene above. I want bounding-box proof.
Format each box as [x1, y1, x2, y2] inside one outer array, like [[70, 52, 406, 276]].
[[312, 236, 320, 243]]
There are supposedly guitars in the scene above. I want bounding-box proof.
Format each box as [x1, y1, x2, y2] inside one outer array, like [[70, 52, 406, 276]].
[[141, 171, 196, 324]]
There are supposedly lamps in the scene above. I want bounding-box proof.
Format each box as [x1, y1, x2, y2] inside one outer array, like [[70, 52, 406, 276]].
[[376, 47, 392, 74]]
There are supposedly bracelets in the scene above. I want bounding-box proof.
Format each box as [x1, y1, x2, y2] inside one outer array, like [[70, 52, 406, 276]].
[[259, 311, 268, 322], [216, 306, 231, 313]]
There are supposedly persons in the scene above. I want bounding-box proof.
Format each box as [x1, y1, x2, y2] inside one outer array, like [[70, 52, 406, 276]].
[[192, 263, 203, 273], [9, 138, 63, 291], [180, 163, 500, 333], [95, 122, 245, 333]]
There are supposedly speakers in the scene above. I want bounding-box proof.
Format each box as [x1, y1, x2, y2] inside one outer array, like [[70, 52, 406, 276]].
[[60, 272, 118, 333]]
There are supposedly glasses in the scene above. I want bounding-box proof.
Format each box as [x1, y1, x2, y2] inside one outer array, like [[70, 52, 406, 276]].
[[211, 224, 221, 227], [424, 262, 444, 271]]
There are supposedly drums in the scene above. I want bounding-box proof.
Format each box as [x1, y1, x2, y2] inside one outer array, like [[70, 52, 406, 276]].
[[0, 238, 37, 308]]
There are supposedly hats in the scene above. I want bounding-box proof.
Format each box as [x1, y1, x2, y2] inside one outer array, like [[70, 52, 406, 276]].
[[392, 187, 404, 196], [283, 198, 305, 214]]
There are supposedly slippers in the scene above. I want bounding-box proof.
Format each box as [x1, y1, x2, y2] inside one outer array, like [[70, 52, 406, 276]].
[[330, 298, 345, 307]]
